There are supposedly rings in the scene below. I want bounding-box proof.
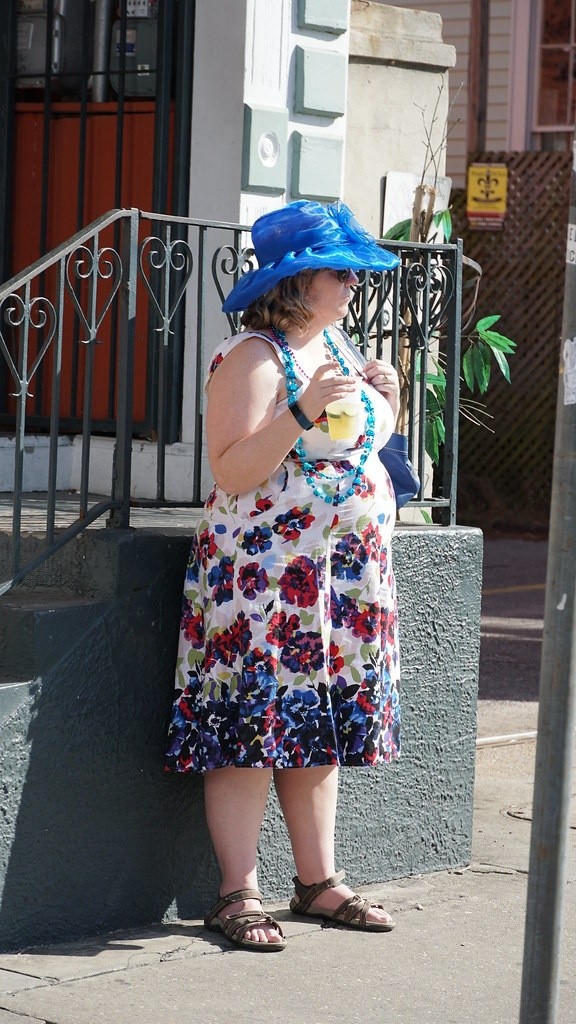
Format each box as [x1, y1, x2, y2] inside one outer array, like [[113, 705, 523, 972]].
[[383, 374, 388, 384]]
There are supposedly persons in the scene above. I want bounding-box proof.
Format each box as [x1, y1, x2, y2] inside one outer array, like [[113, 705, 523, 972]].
[[166, 199, 405, 952]]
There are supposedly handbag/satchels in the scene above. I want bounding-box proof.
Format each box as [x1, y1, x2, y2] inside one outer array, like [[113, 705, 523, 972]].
[[378, 433, 421, 510]]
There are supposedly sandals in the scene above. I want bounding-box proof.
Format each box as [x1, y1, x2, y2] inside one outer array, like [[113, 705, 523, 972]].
[[204, 889, 287, 951], [289, 870, 396, 932]]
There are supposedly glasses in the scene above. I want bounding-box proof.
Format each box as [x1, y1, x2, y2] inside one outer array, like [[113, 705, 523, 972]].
[[316, 267, 359, 283]]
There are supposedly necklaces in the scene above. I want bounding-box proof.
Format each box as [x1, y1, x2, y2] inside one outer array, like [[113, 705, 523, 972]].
[[275, 325, 375, 505], [270, 328, 346, 383]]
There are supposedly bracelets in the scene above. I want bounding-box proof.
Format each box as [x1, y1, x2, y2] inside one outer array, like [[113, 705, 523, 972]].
[[286, 401, 315, 431]]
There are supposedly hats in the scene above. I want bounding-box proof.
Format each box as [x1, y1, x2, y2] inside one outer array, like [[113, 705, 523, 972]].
[[221, 199, 400, 313]]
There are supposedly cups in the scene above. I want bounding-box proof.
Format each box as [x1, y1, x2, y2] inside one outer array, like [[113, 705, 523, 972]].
[[326, 377, 361, 439]]
